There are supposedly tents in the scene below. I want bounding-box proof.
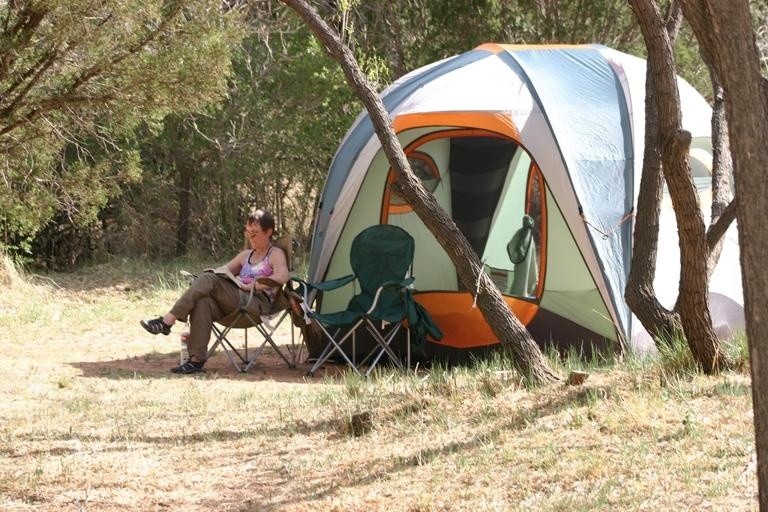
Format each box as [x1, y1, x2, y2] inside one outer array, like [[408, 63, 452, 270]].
[[297, 42, 745, 364]]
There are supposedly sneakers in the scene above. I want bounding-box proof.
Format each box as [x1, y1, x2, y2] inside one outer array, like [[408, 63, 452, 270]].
[[139, 316, 171, 336], [170, 359, 206, 374]]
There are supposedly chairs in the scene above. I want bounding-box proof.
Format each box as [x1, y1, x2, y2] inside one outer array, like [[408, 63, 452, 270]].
[[189, 235, 294, 376], [285, 225, 442, 378]]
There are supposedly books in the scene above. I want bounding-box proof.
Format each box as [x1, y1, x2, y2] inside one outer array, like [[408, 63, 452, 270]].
[[176, 264, 243, 290]]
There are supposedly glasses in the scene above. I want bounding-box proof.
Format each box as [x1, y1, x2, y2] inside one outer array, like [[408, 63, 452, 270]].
[[243, 231, 258, 238]]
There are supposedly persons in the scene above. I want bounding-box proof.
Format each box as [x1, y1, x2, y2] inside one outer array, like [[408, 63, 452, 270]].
[[138, 207, 292, 375]]
